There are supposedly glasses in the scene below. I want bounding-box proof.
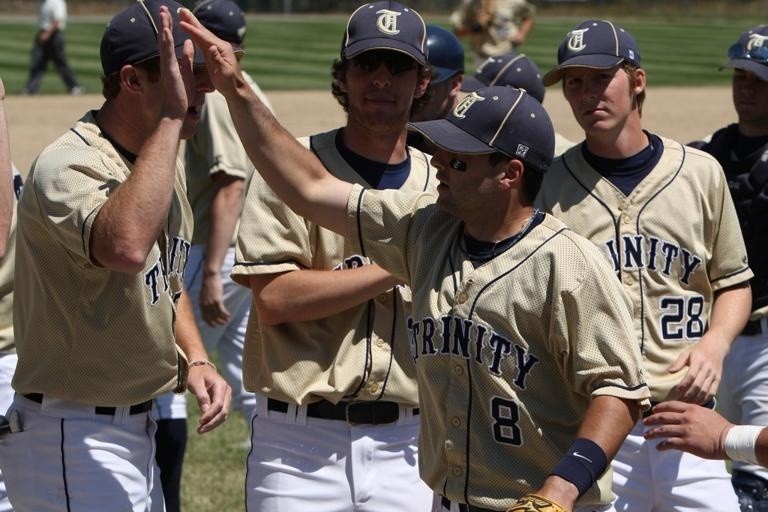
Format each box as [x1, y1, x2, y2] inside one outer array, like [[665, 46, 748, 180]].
[[355, 51, 413, 73]]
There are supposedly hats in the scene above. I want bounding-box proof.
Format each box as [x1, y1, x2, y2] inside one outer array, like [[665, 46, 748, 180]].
[[407, 86, 555, 171], [423, 25, 465, 84], [460, 52, 544, 103], [102, 1, 245, 73], [718, 24, 767, 80], [542, 20, 640, 87], [344, 2, 429, 67]]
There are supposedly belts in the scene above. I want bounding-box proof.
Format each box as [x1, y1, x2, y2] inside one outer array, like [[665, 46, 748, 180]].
[[24, 392, 152, 414], [739, 320, 761, 336], [268, 398, 423, 423]]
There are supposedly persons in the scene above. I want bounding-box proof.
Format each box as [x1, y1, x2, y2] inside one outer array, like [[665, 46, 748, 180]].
[[0, 1, 768, 512]]
